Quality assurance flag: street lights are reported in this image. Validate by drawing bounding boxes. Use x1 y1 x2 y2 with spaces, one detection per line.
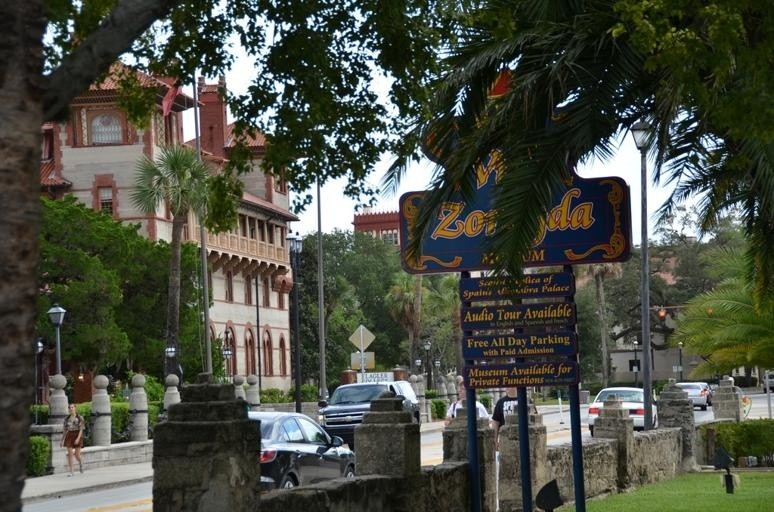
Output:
161 342 179 374
43 299 69 379
284 226 307 416
32 339 46 427
677 341 683 382
415 339 442 392
626 112 661 432
633 340 640 386
218 344 233 385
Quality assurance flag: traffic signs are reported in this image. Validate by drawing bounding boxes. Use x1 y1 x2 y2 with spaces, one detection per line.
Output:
450 276 581 390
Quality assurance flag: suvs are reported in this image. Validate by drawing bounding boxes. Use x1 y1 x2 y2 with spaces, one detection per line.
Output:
316 380 421 452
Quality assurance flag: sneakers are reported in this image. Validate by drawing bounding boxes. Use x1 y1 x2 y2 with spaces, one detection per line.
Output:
79 465 83 471
67 474 75 477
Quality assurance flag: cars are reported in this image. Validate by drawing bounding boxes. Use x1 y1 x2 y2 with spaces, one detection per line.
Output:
760 370 774 393
585 381 659 437
673 378 723 410
242 408 356 492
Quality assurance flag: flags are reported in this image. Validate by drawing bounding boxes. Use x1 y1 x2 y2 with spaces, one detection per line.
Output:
162 75 184 116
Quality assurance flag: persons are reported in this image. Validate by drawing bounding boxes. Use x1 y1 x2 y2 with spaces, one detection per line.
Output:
443 382 489 426
61 404 85 477
492 387 538 450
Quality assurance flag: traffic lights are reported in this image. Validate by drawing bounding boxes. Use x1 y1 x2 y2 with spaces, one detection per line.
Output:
658 309 668 329
